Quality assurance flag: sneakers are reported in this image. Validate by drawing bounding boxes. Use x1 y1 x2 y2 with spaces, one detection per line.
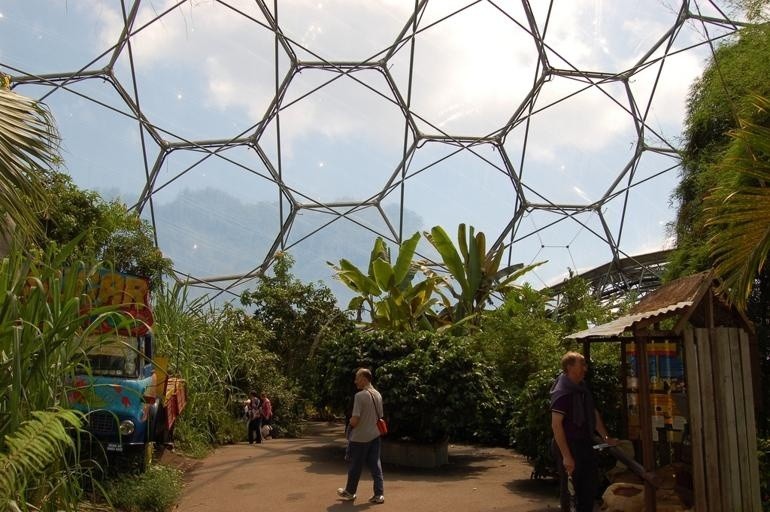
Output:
369 495 385 503
337 488 356 500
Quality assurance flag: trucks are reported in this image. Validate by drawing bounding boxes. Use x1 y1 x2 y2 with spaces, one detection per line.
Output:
16 265 189 477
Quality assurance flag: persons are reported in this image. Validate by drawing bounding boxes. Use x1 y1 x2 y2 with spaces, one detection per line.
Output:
337 367 389 503
243 391 262 445
259 391 274 440
547 352 623 511
223 384 231 409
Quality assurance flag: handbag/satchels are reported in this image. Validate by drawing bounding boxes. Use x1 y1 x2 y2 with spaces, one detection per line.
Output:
376 419 388 434
261 425 270 438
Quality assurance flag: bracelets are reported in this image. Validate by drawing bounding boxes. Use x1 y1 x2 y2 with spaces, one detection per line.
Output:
603 432 608 440
